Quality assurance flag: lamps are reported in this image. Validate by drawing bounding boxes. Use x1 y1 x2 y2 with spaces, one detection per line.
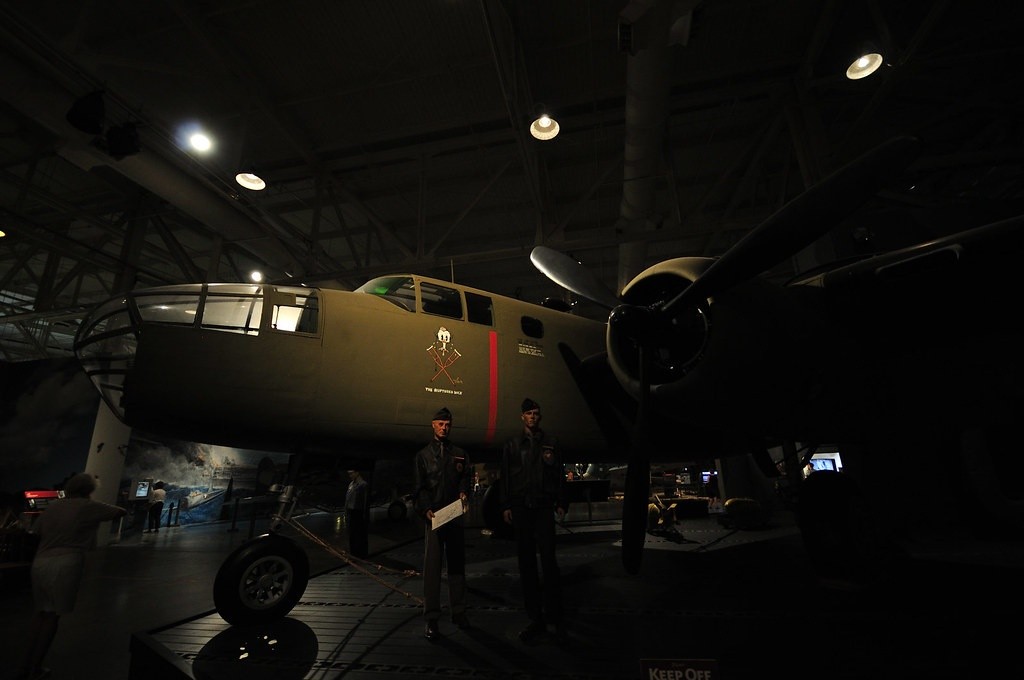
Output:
846 54 882 79
530 117 560 140
235 173 266 191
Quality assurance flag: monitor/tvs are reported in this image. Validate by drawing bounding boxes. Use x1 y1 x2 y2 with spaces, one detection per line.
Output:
810 459 836 471
702 474 709 482
136 481 150 498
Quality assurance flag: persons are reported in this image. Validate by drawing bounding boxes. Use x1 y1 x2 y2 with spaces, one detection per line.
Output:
415 406 472 639
567 463 585 481
806 463 816 477
143 481 166 533
20 472 127 680
707 468 719 509
345 465 368 562
500 397 571 645
646 497 672 529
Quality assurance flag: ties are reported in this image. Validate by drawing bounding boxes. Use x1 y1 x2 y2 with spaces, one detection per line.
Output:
440 442 445 459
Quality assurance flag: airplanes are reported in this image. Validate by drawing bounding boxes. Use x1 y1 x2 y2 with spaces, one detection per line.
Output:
0 132 1023 629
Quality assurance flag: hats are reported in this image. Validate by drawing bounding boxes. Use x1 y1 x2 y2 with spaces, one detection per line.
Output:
520 398 539 413
433 408 452 420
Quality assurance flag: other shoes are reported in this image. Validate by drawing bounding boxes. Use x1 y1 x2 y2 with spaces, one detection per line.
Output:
154 530 159 533
518 620 547 641
556 622 571 645
424 620 440 641
450 610 466 626
29 667 52 680
143 530 153 533
